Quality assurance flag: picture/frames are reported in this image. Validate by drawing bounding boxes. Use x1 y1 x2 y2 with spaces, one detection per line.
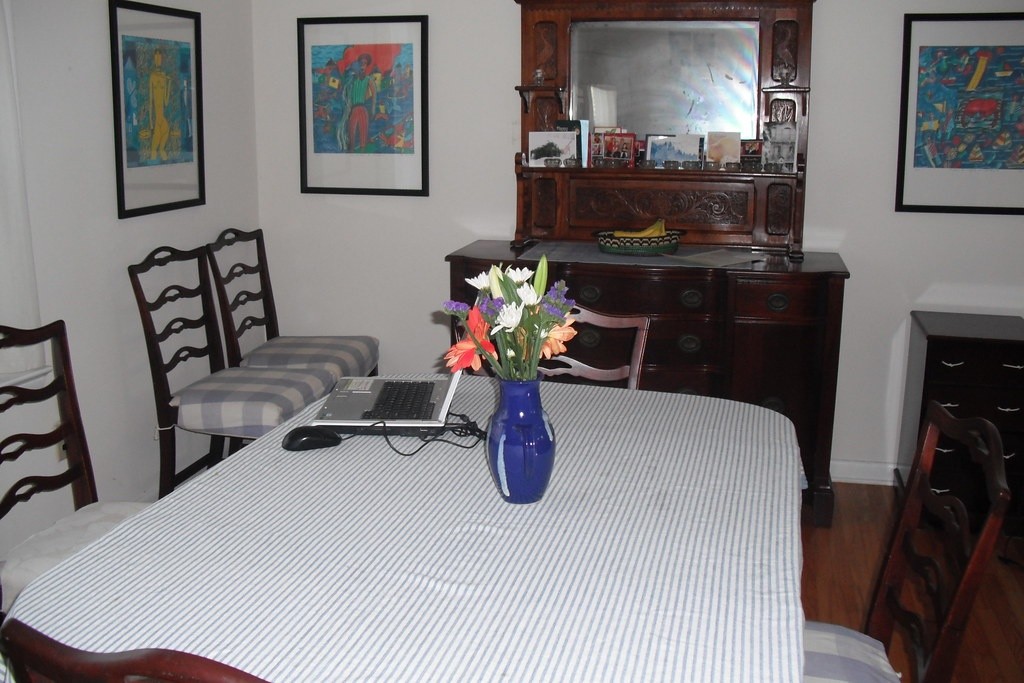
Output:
644 135 705 168
108 1 208 221
600 131 636 160
294 16 428 195
895 12 1024 219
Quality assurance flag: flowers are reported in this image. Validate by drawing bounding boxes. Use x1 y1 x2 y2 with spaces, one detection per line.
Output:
439 252 581 385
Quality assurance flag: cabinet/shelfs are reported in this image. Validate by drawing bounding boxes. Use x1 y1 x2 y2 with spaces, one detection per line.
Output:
889 310 1023 561
446 240 850 528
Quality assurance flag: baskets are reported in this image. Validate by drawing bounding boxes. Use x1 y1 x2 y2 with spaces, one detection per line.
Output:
591 227 688 257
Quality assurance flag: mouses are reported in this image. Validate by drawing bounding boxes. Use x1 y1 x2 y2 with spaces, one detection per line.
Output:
282 425 341 451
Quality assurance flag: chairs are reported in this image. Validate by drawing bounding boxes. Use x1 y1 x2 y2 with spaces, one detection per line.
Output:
538 301 649 394
803 401 1009 683
129 225 385 500
0 319 155 622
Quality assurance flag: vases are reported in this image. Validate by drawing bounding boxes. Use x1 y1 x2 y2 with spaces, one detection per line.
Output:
484 371 556 503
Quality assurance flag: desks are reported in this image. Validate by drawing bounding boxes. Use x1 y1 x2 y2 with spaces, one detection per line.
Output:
0 374 809 682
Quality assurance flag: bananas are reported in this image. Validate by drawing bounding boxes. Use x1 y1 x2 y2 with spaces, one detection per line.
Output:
612 218 667 236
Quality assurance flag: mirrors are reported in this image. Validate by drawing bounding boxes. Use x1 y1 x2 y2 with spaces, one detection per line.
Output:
571 20 760 146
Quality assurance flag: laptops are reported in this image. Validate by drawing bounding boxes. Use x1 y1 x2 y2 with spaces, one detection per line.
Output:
312 290 491 436
